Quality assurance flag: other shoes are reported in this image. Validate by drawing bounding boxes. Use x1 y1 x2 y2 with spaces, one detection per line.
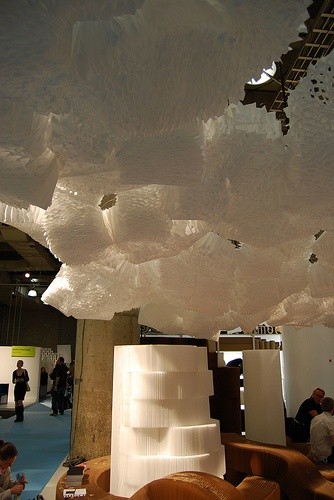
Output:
50 412 64 416
14 418 24 422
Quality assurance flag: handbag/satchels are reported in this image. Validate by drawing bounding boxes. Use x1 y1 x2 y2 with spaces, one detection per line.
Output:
26 382 30 391
63 396 72 411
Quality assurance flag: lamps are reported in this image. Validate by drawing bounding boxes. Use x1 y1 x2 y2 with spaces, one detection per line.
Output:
27 286 37 297
246 59 277 86
25 264 31 278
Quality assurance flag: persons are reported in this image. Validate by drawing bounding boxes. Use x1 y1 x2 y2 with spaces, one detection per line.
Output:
12 360 30 422
309 396 334 464
39 367 48 398
50 356 68 416
289 387 326 443
0 439 25 500
49 361 62 410
